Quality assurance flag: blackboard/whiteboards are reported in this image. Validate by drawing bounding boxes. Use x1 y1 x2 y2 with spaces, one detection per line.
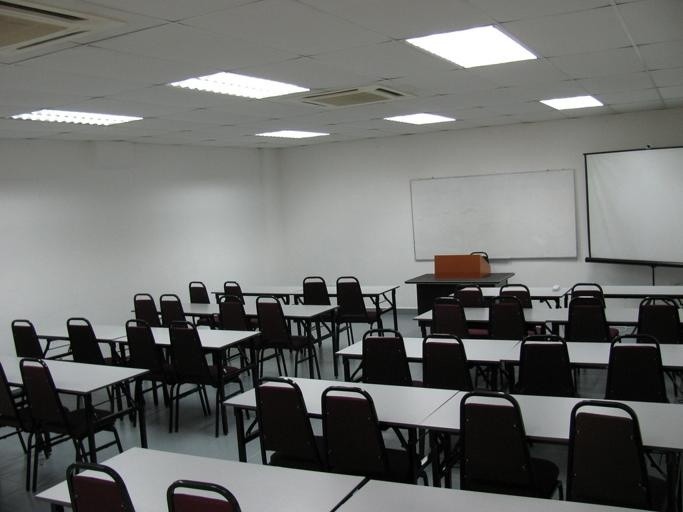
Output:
410 168 579 261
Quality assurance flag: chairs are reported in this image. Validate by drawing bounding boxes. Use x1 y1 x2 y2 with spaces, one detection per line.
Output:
4 275 680 509
